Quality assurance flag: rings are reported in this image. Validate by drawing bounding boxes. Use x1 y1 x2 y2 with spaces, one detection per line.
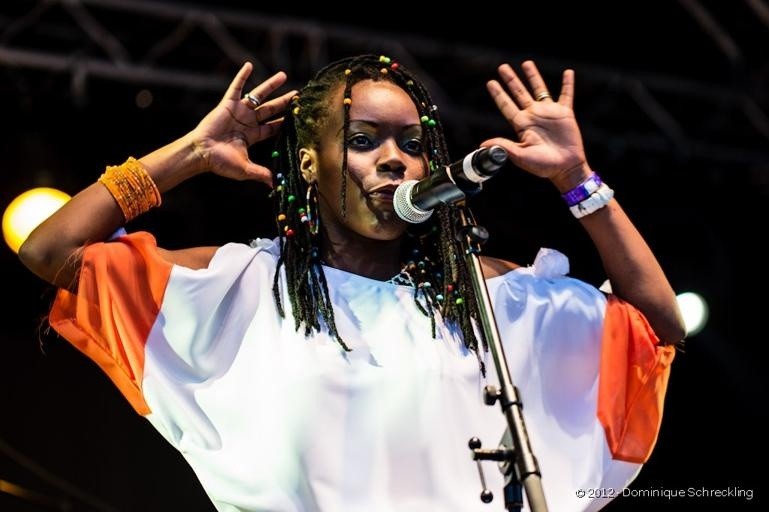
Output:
243 91 261 107
535 91 552 101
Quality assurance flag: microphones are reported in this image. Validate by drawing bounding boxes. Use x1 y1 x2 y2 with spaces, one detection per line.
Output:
392 145 508 224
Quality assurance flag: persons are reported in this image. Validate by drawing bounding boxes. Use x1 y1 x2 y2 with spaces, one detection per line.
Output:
16 55 689 512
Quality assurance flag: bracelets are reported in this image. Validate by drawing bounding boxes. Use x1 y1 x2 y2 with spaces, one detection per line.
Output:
559 169 616 220
96 151 166 223
